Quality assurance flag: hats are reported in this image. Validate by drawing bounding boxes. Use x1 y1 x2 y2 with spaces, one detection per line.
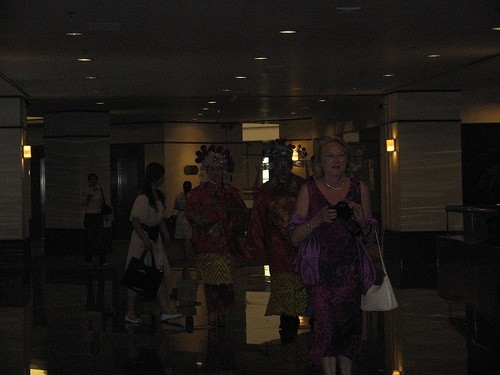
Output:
195 145 233 187
255 138 310 179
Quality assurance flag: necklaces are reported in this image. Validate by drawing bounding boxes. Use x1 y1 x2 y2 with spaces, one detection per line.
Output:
320 175 346 190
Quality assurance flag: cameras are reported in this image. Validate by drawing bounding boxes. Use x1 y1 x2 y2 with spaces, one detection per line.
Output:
329 201 353 221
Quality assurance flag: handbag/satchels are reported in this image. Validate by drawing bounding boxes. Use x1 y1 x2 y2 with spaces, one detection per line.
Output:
358 274 399 311
126 247 164 297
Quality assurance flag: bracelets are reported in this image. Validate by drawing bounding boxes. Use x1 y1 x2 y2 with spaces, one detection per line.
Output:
305 223 315 231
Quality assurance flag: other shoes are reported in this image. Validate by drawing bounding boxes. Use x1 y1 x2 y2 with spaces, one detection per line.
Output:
161 312 184 321
123 313 141 324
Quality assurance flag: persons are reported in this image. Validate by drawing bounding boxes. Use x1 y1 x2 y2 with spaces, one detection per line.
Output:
287 136 376 375
123 164 183 324
245 140 323 345
173 181 192 253
81 173 111 267
184 144 250 327
348 144 378 192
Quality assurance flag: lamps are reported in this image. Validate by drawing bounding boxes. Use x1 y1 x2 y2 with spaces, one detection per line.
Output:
23 145 32 159
386 139 395 152
